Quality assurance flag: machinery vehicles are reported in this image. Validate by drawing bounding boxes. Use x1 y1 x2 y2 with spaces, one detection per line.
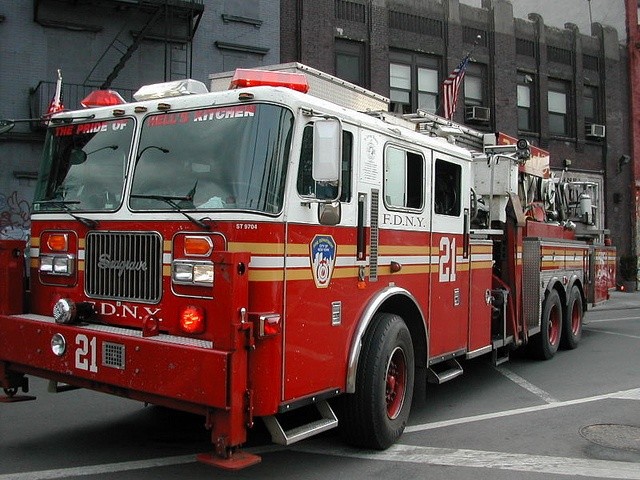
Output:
0 35 624 472
